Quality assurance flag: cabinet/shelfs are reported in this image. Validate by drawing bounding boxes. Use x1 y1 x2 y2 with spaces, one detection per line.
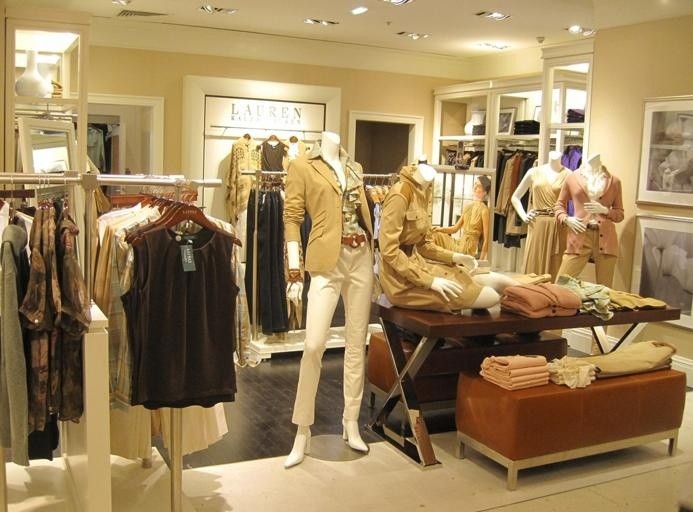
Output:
3 5 87 173
431 69 589 273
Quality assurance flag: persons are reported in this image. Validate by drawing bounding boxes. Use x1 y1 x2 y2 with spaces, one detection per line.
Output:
378 163 522 316
554 153 624 356
282 128 375 468
510 151 573 281
429 175 490 261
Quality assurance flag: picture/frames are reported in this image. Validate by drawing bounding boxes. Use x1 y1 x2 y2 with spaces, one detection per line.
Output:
628 95 693 330
497 107 517 135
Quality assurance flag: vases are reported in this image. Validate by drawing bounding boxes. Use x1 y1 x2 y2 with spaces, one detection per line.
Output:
15 50 50 98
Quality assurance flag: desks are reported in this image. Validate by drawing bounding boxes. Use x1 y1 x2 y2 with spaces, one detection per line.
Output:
363 292 681 472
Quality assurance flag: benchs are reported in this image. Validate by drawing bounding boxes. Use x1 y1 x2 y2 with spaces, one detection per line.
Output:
365 332 569 431
456 351 685 487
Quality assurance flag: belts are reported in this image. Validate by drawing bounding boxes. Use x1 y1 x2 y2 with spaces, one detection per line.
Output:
587 223 600 231
342 234 369 250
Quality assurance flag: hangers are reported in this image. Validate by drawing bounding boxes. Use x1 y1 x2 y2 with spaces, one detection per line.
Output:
123 182 242 250
6 174 71 233
242 129 297 144
